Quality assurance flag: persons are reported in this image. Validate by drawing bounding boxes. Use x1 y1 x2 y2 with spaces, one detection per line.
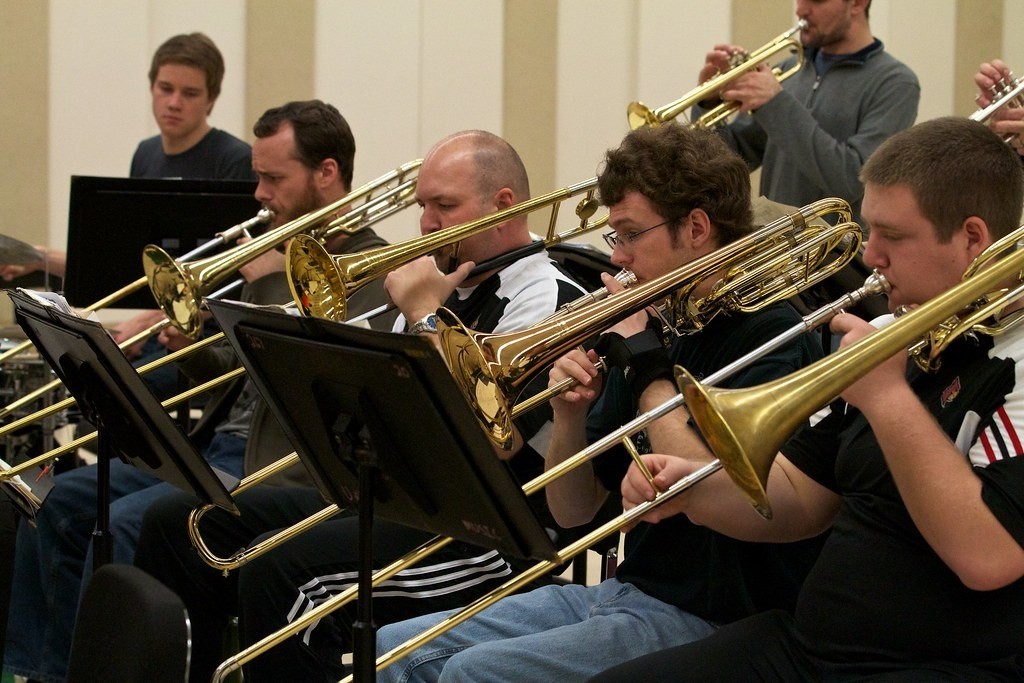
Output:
1 99 409 683
589 116 1024 683
377 119 828 681
228 127 613 682
691 0 923 322
2 31 260 288
973 56 1024 161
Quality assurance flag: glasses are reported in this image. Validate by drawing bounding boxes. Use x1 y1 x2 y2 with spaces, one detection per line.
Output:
603 214 688 250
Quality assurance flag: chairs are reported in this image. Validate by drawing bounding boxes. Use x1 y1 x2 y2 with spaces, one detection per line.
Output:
64 562 192 683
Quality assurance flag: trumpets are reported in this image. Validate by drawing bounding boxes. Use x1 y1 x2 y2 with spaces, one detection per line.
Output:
967 69 1024 145
626 20 809 132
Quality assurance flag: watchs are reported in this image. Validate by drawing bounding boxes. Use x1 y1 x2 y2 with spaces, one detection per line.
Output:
407 314 437 335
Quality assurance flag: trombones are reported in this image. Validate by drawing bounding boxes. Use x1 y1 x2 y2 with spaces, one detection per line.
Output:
0 175 611 480
0 155 424 420
210 219 1023 683
187 196 864 578
